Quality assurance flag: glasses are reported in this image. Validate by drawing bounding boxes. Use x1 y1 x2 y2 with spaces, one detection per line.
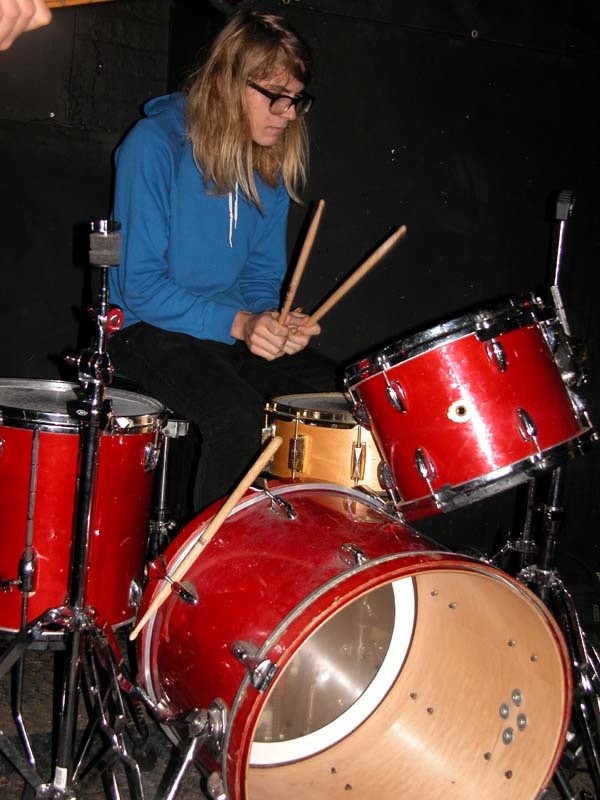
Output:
246 79 313 116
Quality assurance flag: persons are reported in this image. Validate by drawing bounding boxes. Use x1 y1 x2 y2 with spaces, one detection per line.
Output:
106 9 333 515
0 0 175 375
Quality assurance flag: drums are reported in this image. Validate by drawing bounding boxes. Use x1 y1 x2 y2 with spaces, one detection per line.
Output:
130 478 574 800
343 291 600 525
260 393 397 507
0 376 171 638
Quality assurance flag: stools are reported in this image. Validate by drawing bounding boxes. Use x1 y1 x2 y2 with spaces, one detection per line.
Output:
106 372 191 561
